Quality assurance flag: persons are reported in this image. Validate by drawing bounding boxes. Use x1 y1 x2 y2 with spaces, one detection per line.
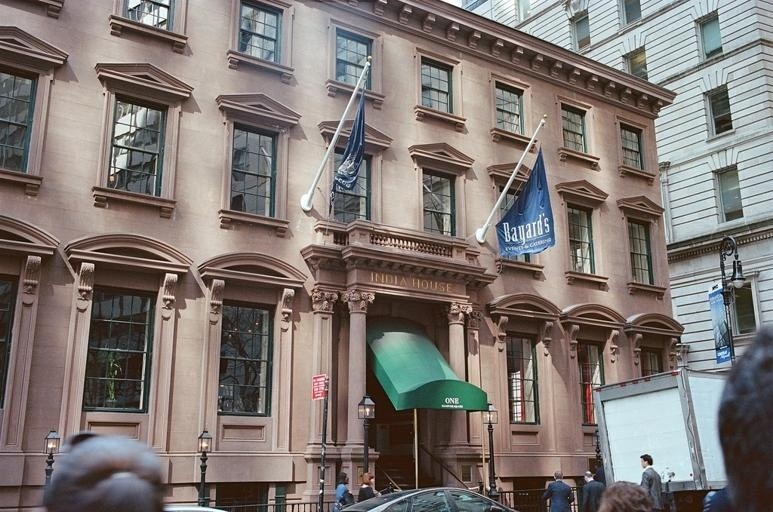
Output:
701 484 732 512
540 470 574 512
638 454 665 512
580 471 605 511
39 433 168 512
333 472 351 512
714 324 772 512
598 480 651 512
356 471 378 504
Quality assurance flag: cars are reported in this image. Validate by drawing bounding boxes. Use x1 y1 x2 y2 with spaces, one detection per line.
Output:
335 484 520 512
162 503 228 511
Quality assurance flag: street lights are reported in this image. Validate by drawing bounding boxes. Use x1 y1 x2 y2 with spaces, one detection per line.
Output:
479 401 499 506
41 426 61 508
717 233 745 368
355 393 377 500
195 430 213 507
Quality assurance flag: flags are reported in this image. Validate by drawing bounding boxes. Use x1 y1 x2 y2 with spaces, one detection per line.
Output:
493 144 555 259
328 81 367 218
